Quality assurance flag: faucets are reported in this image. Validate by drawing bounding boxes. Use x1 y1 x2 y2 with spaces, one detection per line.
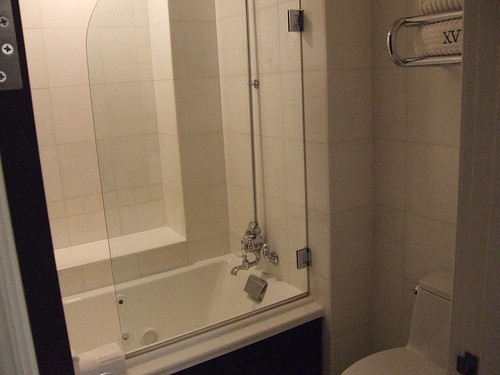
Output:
231 254 261 275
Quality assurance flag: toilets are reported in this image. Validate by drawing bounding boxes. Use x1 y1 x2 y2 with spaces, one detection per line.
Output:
340 262 453 375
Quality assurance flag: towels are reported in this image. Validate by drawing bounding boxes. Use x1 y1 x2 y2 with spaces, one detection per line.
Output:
70 342 127 375
410 0 464 57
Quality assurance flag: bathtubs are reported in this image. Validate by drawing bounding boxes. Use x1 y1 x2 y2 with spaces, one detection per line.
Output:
60 253 307 363
